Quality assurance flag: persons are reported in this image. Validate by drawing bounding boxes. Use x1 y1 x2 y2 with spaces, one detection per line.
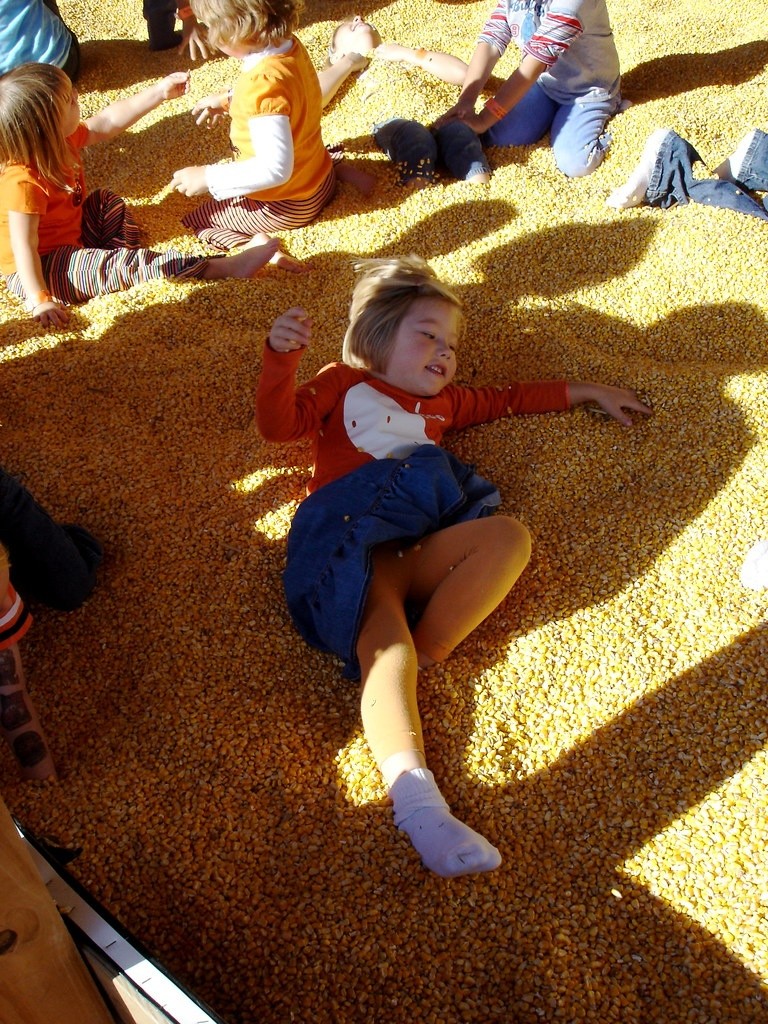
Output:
0 468 100 783
254 254 654 877
0 0 768 328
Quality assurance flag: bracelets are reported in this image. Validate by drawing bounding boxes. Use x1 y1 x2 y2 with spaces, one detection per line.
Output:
484 98 507 120
